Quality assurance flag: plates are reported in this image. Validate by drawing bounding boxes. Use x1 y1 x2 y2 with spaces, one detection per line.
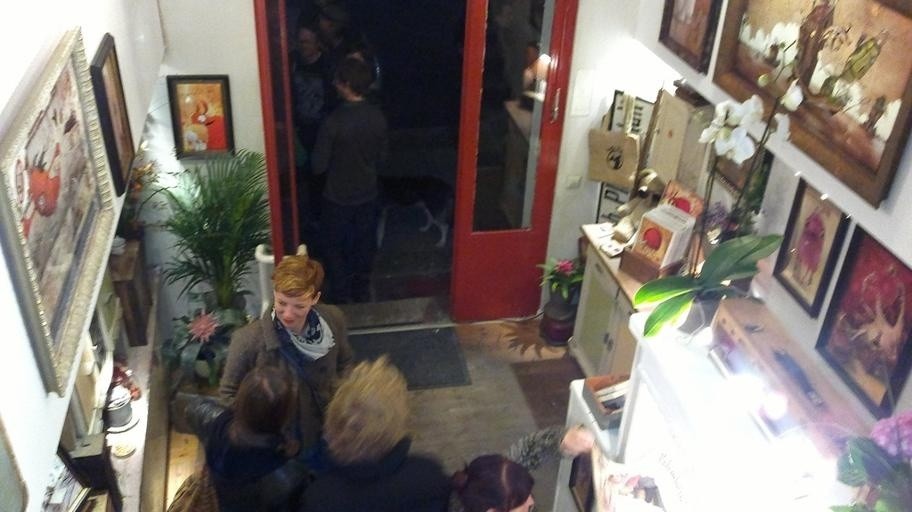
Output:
102 413 139 435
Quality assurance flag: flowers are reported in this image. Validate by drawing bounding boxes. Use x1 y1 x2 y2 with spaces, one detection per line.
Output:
127 140 161 203
160 303 248 390
828 407 912 512
535 253 587 309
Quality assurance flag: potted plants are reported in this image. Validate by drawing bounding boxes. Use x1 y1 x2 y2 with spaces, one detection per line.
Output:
147 144 277 318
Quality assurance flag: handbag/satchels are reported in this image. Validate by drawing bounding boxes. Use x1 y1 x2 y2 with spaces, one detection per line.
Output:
165 472 220 511
587 95 640 193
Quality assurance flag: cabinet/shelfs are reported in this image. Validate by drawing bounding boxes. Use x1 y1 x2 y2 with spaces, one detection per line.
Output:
109 220 155 348
565 222 648 383
499 101 534 229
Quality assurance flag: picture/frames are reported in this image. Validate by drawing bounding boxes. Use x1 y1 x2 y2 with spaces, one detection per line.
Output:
87 31 139 202
566 423 596 511
164 70 239 159
1 24 119 396
0 417 29 512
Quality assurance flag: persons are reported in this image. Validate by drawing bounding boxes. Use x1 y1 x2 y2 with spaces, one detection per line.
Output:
171 366 312 512
451 423 594 511
220 255 356 427
278 0 542 303
301 352 447 510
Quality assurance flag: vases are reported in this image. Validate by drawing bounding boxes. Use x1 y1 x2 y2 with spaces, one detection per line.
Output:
540 299 576 346
165 381 226 435
121 196 145 240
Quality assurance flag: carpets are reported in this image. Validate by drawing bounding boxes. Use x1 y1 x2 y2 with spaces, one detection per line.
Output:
343 322 474 392
510 353 588 430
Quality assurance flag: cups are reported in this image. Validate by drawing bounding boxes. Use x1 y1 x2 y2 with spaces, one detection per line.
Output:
109 235 127 255
104 388 133 427
